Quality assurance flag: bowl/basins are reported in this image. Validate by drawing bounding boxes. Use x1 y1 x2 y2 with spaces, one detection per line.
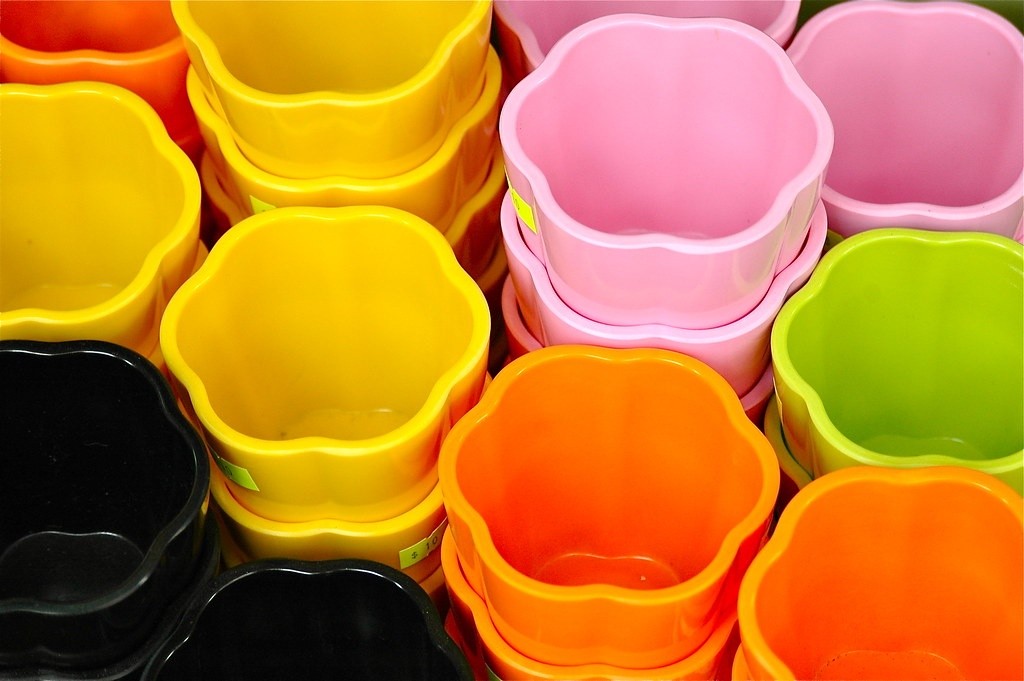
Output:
479 234 506 294
733 644 757 681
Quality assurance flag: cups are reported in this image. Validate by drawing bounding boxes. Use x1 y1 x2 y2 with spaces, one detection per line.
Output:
439 344 780 669
772 228 1024 494
0 512 222 681
202 149 502 248
171 372 491 582
170 0 490 178
496 189 827 397
440 524 740 681
0 0 197 163
160 208 491 521
139 559 474 681
0 341 209 653
0 81 206 356
500 14 834 328
502 274 774 422
764 397 812 490
494 0 799 67
737 467 1024 681
214 514 446 602
785 0 1024 244
187 46 501 207
